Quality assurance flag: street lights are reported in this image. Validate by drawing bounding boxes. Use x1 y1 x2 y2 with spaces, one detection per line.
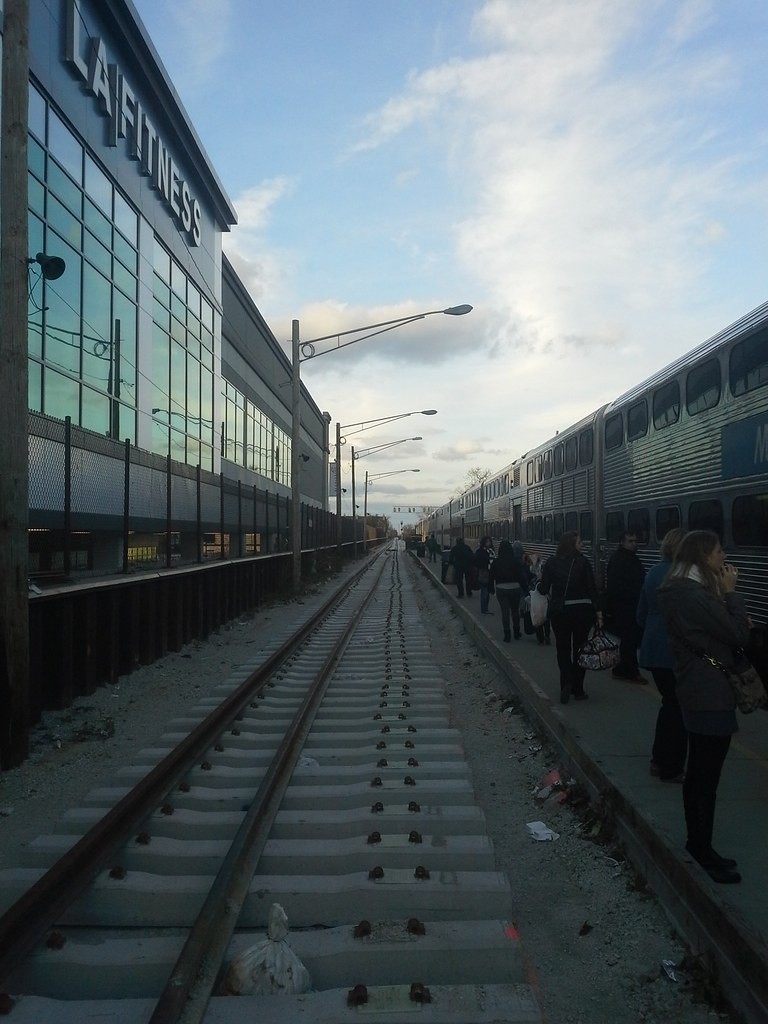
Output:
292 306 474 600
351 437 423 561
363 469 420 555
335 408 438 571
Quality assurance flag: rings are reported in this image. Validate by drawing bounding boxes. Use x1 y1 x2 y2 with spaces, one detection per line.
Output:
732 572 737 575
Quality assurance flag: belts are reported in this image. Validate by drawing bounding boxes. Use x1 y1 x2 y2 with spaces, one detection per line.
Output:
565 599 592 605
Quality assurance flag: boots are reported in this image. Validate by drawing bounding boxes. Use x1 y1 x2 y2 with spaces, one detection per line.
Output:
682 783 740 884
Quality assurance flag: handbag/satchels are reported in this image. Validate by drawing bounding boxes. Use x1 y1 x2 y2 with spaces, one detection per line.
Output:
578 626 621 671
529 583 548 627
524 602 535 634
727 663 767 715
446 563 455 583
546 591 565 616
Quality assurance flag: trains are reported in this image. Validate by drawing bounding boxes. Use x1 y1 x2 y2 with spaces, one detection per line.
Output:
415 302 768 651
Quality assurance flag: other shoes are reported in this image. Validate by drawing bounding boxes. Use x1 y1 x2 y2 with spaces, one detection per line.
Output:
515 633 522 640
574 692 590 700
482 611 494 616
504 637 510 643
611 668 649 685
561 695 569 703
651 763 686 783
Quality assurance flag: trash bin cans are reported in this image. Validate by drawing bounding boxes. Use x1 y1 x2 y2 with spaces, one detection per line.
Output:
417 541 425 558
440 549 456 586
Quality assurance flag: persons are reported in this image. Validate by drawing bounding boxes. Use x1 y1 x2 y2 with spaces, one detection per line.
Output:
449 532 648 706
637 528 749 885
425 534 437 563
